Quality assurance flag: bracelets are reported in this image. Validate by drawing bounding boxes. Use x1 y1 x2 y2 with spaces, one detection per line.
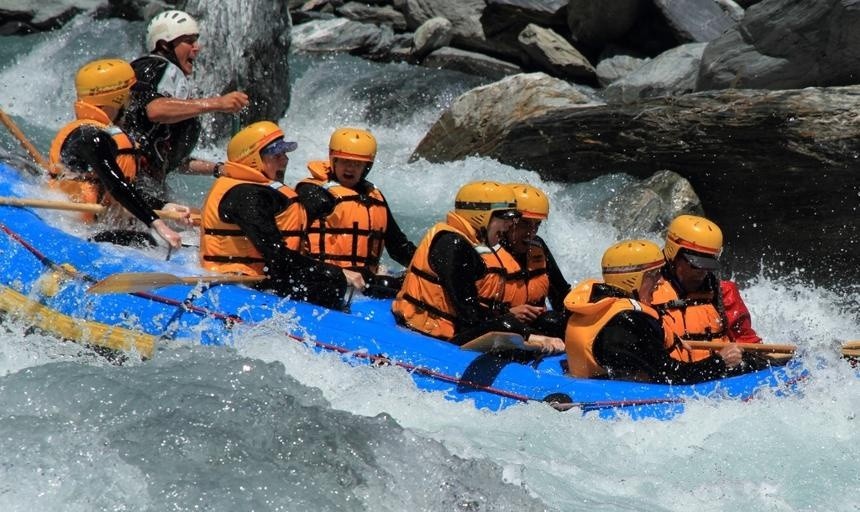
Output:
212 162 225 177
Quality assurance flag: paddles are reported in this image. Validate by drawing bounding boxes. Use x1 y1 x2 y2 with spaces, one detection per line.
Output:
85 273 266 293
460 331 543 351
684 340 860 356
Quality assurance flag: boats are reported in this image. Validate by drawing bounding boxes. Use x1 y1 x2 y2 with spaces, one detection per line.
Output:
0 154 815 423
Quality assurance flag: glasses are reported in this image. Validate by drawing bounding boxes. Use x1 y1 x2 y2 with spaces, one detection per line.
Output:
647 269 661 281
178 36 197 44
516 219 541 231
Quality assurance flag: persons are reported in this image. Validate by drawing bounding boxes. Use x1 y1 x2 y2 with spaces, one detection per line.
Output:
117 9 249 231
293 125 417 297
201 121 347 308
648 213 786 378
491 182 572 341
47 58 195 266
391 181 564 365
561 240 742 388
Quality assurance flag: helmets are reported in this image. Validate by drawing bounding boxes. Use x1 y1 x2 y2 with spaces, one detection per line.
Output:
146 10 199 52
75 59 137 109
601 215 723 293
455 182 549 231
227 120 297 172
329 128 376 178
